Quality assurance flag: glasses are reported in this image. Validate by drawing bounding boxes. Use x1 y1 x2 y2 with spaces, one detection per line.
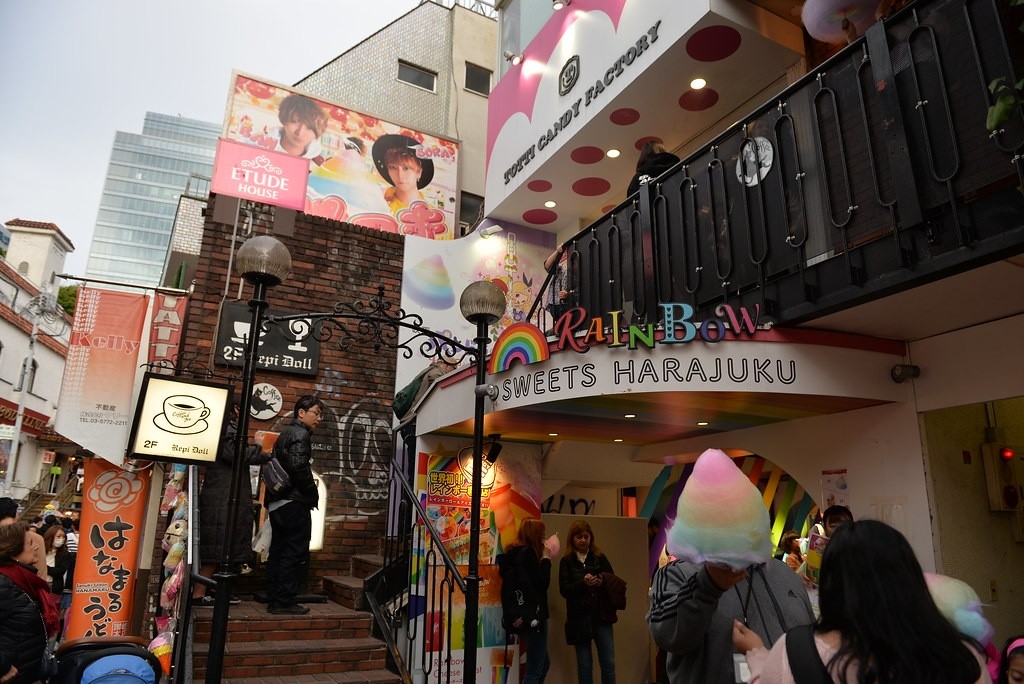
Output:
304 408 323 420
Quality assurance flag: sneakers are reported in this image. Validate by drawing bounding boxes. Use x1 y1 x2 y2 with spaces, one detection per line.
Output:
192 596 215 609
205 588 241 604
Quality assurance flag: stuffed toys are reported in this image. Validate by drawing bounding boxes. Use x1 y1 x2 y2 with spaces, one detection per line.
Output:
148 462 188 676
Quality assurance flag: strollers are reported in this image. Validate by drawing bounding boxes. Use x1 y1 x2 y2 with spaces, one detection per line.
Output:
51 635 162 684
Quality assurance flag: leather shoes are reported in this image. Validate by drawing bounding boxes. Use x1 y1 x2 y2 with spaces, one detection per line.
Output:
267 600 311 614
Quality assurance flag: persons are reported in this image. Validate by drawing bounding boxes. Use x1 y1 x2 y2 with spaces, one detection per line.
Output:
841 0 914 45
196 377 275 607
262 394 325 616
744 119 772 143
558 519 628 684
995 634 1024 684
498 516 552 684
542 242 583 325
780 530 807 573
626 141 681 223
645 551 819 684
797 504 856 622
0 497 80 684
236 93 446 236
647 514 662 551
731 519 992 684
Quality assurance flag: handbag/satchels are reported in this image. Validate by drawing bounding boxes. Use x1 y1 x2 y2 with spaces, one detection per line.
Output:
564 614 593 645
260 428 293 494
501 547 549 633
42 652 59 675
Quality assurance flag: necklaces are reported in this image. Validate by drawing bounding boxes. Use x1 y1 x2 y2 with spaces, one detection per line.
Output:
734 564 754 629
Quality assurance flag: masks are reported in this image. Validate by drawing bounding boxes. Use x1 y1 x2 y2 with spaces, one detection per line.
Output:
52 537 65 548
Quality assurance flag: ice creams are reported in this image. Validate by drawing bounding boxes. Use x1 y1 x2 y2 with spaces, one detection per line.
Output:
149 637 172 675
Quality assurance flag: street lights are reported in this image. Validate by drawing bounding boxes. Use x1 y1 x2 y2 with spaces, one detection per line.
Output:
460 280 507 684
203 234 293 684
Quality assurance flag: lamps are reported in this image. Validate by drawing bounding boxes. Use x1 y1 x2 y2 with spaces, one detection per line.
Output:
552 0 571 10
487 433 503 462
504 50 524 66
475 383 498 400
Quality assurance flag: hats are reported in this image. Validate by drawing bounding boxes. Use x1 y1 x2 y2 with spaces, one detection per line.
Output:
371 134 434 191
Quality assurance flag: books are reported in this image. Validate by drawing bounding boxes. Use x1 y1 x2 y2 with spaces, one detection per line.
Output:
805 532 832 591
